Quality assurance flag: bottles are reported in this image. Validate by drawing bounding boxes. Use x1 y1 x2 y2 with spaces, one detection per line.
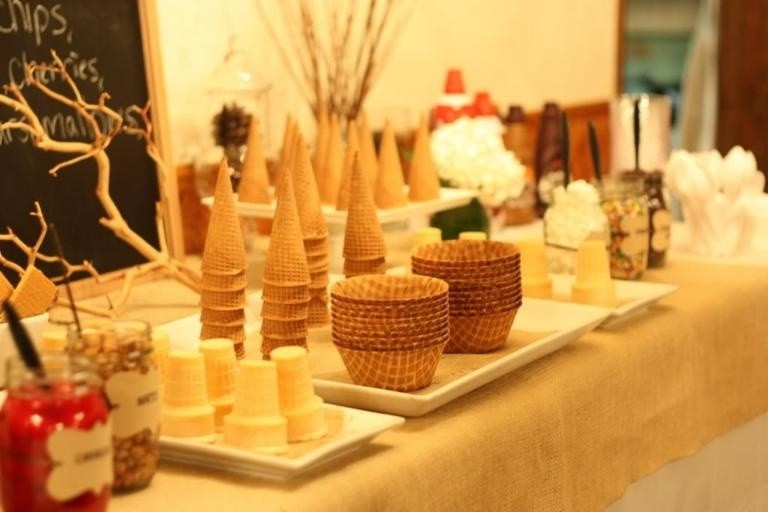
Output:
536 103 566 218
1 351 113 510
504 106 536 226
64 317 162 494
544 173 672 280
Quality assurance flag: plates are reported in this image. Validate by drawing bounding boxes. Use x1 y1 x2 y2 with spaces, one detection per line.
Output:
158 399 406 480
522 274 678 328
201 187 475 223
153 272 609 418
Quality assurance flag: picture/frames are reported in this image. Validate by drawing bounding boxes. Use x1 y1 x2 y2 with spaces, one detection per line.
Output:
1 2 187 318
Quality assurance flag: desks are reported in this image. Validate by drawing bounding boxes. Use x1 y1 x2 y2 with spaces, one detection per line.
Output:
203 186 476 247
